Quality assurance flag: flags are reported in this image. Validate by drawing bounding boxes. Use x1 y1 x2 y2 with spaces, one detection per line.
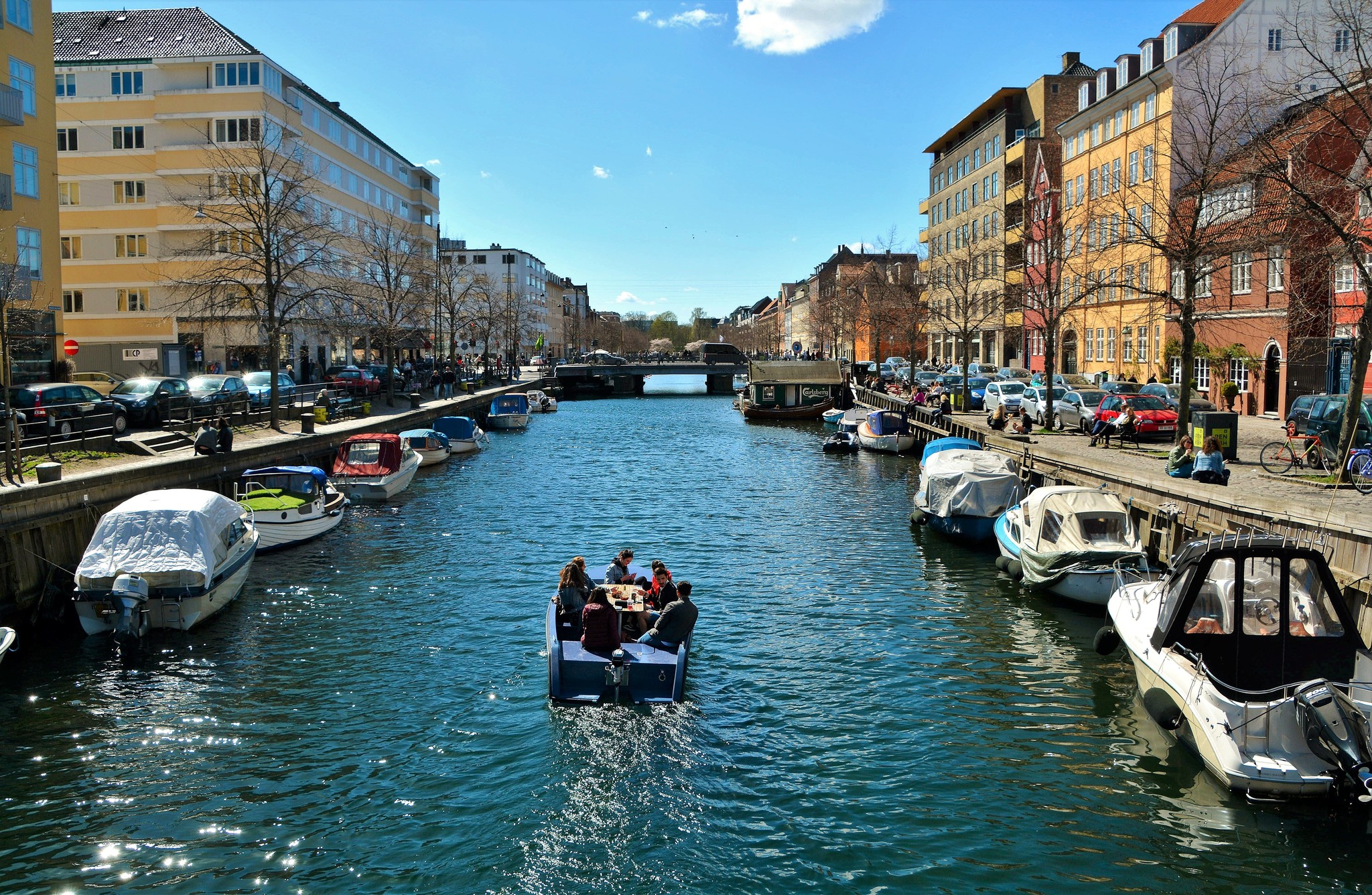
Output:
535 334 542 350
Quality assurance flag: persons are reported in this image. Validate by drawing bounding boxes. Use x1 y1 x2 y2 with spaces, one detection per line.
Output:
558 549 698 652
638 348 692 365
1117 373 1126 381
931 354 937 366
402 352 526 399
1146 372 1157 384
540 350 598 366
1165 435 1194 478
904 380 952 428
1013 406 1032 435
936 358 963 374
1085 404 1138 449
988 403 1012 432
194 416 233 456
1202 392 1208 401
1192 435 1231 486
318 389 336 420
286 359 324 384
1042 376 1046 385
1128 373 1137 383
755 348 827 361
862 375 889 399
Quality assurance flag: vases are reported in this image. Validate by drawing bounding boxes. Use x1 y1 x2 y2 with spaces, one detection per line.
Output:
77 404 96 412
49 417 55 426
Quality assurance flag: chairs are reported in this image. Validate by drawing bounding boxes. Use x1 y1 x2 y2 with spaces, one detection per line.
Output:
1329 409 1340 421
1077 394 1090 405
1289 620 1311 636
1186 618 1226 635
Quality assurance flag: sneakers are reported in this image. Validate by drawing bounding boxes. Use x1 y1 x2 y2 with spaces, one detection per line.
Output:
623 622 637 631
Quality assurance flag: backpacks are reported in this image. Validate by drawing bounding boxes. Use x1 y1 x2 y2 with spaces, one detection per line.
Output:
987 411 994 425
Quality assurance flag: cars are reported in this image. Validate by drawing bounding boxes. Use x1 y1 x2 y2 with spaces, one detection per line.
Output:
833 356 1217 443
187 364 252 419
333 369 381 397
556 359 567 365
241 371 296 411
530 356 543 366
583 353 626 366
106 376 195 428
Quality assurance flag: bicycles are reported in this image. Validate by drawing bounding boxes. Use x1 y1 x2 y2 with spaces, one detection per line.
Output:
501 363 508 372
473 362 485 371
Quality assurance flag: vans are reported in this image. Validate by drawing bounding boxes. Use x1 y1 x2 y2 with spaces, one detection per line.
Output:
700 342 748 365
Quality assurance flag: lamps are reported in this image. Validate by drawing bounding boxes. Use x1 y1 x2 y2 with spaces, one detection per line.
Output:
801 285 805 292
557 302 561 307
785 298 789 302
1122 324 1132 334
453 269 458 276
193 188 207 219
540 291 545 297
372 242 379 256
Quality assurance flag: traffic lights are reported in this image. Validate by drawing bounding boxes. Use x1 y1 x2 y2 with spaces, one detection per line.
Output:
454 340 459 348
591 338 599 346
495 341 499 348
468 339 476 347
516 340 519 349
471 322 474 328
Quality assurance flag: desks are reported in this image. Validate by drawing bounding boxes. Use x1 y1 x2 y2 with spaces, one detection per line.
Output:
600 583 643 638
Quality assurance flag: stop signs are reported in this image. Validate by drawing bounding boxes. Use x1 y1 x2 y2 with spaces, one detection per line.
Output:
63 340 79 356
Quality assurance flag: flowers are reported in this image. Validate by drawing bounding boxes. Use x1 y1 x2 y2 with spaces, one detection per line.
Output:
49 413 56 417
82 403 92 406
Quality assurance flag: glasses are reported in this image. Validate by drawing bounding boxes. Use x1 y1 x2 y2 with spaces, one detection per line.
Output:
627 551 634 555
1185 441 1192 444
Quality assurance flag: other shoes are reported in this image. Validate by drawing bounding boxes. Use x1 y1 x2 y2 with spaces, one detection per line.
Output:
1085 431 1093 438
1087 443 1096 447
1102 445 1109 449
1090 435 1099 440
932 421 937 425
938 425 942 429
908 414 912 418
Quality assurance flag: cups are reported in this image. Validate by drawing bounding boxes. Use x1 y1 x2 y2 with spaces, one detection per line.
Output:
1189 452 1195 462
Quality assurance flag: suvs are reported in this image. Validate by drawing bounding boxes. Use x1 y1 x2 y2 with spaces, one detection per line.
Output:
0 383 127 448
323 364 360 387
67 371 127 397
356 364 406 393
1260 393 1372 494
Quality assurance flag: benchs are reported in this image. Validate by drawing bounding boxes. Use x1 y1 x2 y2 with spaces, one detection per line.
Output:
314 396 363 421
1096 415 1143 448
461 377 481 390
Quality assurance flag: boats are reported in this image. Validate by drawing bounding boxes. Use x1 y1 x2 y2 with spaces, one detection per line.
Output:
330 416 481 499
822 405 915 454
486 390 557 429
71 487 261 637
227 466 351 550
912 437 1372 805
547 578 693 703
0 627 16 665
732 358 843 418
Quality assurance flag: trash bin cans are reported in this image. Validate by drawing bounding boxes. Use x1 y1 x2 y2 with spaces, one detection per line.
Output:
1191 412 1239 460
950 383 972 410
